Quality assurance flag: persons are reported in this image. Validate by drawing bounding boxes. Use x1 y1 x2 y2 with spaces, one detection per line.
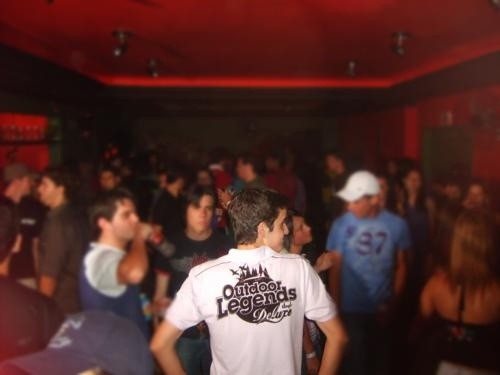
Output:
325 170 412 374
284 207 338 375
0 195 62 375
78 187 162 340
1 142 499 283
0 308 157 375
156 184 234 375
409 209 500 373
149 187 350 375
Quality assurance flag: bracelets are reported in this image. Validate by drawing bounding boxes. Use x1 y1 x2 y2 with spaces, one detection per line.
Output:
305 351 317 359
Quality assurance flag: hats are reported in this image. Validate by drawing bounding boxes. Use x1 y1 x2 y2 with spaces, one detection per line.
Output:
6 310 153 375
336 171 379 203
5 161 40 182
